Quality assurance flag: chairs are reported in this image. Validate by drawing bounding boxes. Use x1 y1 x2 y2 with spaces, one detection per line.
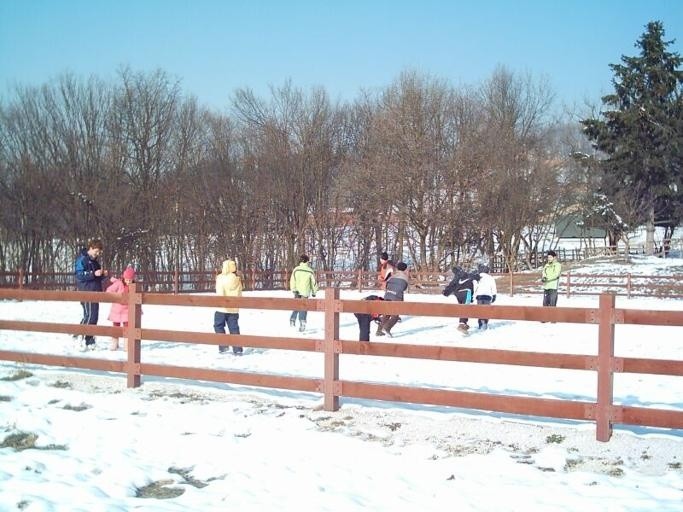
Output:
375 331 385 336
383 327 393 337
88 343 96 350
219 347 229 353
457 324 469 336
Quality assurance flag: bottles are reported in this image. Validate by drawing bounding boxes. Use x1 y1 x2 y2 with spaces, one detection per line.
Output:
124 268 135 281
222 260 237 274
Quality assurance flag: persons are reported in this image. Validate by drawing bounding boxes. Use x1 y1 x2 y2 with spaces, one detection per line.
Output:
472 265 497 329
377 252 402 322
539 251 562 324
376 262 409 337
442 266 482 337
72 239 108 352
354 295 384 341
289 254 320 332
104 264 142 351
214 260 244 354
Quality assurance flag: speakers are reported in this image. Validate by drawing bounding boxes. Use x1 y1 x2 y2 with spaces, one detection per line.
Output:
290 319 295 326
299 320 307 333
123 337 129 352
109 338 119 350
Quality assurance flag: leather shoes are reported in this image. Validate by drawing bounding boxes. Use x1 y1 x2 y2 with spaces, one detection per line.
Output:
492 295 495 302
